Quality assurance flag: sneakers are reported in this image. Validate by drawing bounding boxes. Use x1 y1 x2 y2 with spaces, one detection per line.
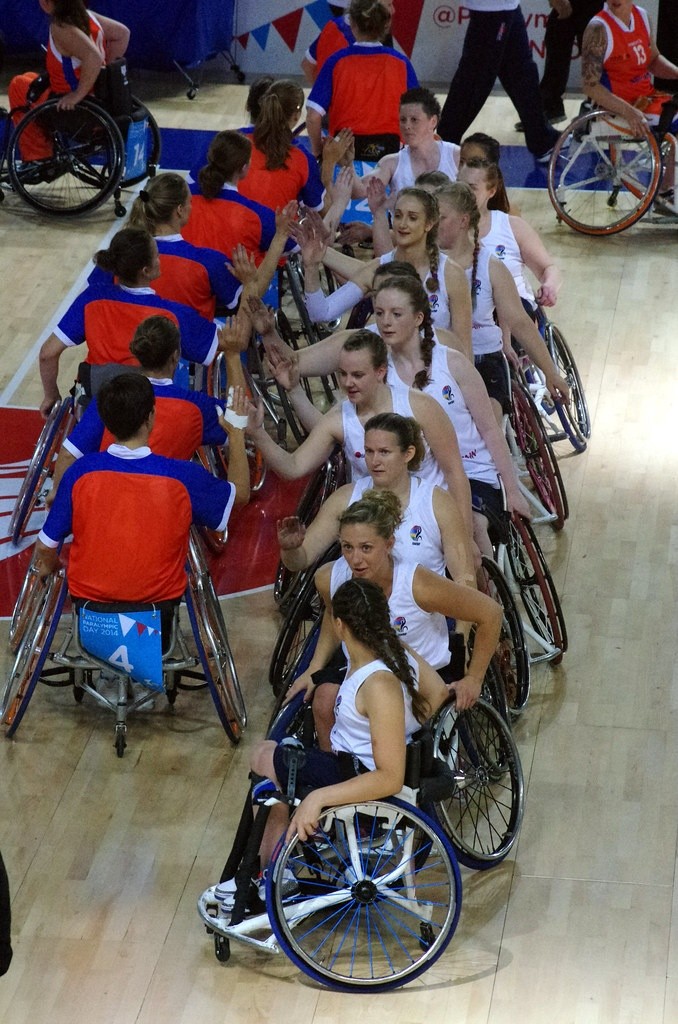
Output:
537 134 575 162
222 871 300 912
213 871 273 900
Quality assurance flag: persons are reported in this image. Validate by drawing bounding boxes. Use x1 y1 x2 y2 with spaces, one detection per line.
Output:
513 0 608 131
437 0 564 167
9 0 130 170
36 71 354 656
213 89 576 919
579 0 678 204
298 1 424 160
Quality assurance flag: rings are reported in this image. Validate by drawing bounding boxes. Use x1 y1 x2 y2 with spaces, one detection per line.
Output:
334 136 340 142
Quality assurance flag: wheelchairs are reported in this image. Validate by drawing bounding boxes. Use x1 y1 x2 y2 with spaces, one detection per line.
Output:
547 96 677 238
202 285 593 994
0 59 162 216
0 189 343 745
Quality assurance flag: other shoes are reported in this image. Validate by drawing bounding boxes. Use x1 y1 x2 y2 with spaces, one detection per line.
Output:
514 104 567 131
